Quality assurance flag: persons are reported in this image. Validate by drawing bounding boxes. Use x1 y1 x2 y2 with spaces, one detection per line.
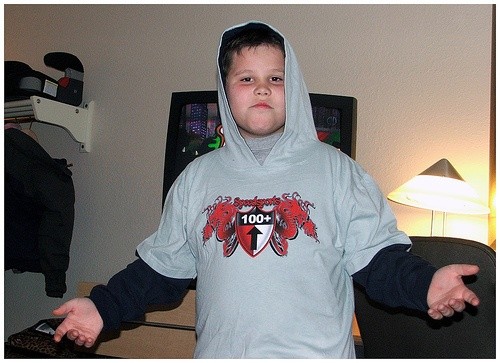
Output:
53 20 479 359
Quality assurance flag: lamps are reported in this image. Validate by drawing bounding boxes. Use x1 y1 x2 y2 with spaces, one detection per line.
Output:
388 158 491 235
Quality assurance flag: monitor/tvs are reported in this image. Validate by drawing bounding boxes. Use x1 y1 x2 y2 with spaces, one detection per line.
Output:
162 90 358 213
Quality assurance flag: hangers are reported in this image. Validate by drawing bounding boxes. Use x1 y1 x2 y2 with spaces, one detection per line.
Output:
4 114 38 144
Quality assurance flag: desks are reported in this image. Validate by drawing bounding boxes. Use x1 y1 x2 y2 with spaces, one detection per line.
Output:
80 281 363 359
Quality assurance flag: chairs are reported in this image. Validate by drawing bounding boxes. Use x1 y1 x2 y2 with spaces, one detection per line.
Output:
353 236 496 357
